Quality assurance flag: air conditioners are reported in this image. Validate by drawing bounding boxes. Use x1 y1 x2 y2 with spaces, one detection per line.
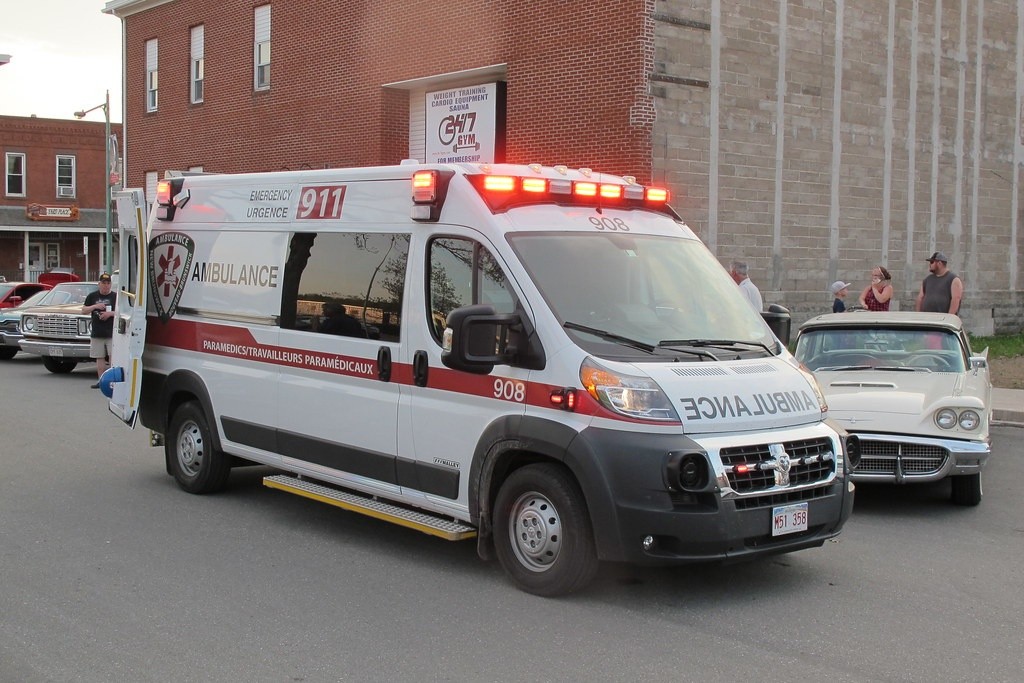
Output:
58 186 75 196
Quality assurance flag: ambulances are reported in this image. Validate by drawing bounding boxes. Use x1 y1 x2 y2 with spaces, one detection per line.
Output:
109 150 863 599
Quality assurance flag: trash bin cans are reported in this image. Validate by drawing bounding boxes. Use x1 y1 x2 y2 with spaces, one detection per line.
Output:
759 303 792 351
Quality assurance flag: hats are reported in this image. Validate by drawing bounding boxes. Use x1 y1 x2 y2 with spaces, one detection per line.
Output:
100 273 111 281
877 264 891 280
832 281 851 295
926 252 948 262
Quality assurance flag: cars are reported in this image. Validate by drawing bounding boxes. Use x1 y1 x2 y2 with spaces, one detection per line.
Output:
779 309 994 504
17 280 115 375
0 289 89 359
0 271 80 308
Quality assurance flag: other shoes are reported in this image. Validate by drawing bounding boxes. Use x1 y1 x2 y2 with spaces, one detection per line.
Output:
91 382 100 389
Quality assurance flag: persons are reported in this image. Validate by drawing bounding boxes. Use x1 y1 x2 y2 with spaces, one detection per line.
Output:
917 252 964 348
831 280 852 350
727 259 763 313
311 302 363 338
81 274 118 388
859 266 893 350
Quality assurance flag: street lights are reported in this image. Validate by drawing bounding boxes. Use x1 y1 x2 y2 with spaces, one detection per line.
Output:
74 88 113 282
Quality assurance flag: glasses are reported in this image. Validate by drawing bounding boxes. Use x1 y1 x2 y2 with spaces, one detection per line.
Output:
930 261 935 264
